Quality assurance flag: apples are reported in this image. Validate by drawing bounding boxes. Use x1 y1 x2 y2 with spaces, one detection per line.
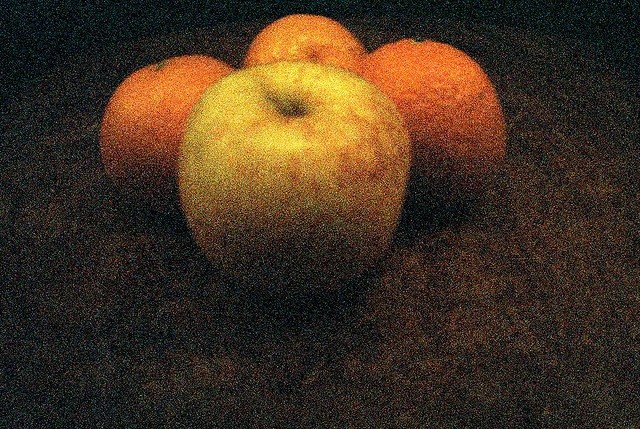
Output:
179 59 413 295
